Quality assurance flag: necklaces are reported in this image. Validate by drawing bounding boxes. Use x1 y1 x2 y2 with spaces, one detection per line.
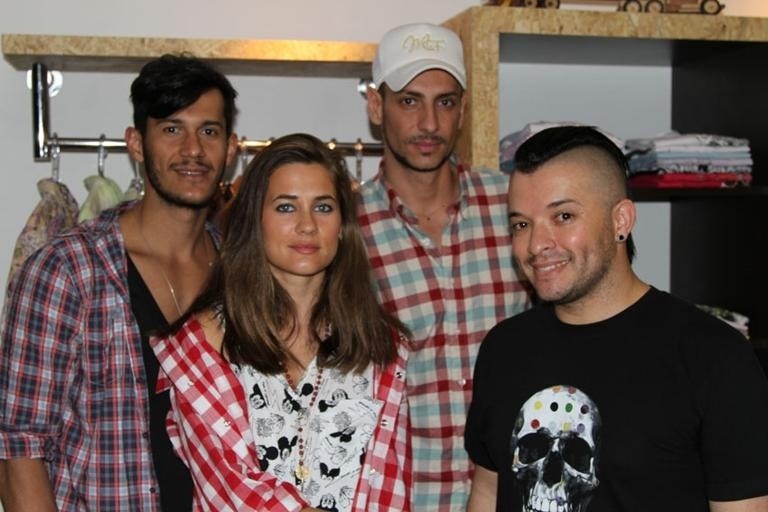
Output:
412 205 449 222
279 317 334 492
132 208 213 319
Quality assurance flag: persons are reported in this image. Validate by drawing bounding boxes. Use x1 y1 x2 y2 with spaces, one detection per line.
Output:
463 129 767 512
149 131 418 512
202 20 542 512
0 50 239 512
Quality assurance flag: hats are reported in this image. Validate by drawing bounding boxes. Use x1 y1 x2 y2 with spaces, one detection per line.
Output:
371 21 467 93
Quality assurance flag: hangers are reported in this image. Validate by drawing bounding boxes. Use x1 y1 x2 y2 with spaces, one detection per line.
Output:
83 132 123 189
48 132 63 189
232 135 252 188
350 136 365 188
129 156 146 189
